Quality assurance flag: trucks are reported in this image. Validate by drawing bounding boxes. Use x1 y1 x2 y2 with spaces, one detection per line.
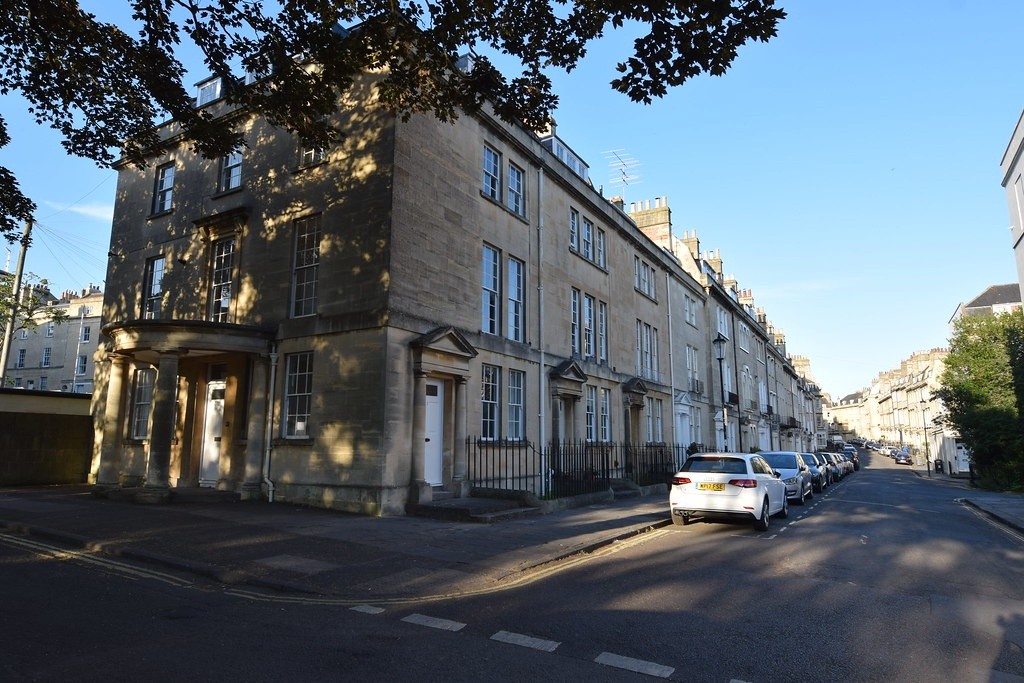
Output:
832 435 843 444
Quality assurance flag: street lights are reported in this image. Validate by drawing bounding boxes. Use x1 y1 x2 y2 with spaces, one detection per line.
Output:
712 333 729 452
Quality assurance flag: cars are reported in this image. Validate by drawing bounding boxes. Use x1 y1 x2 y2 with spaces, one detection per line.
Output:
819 452 854 483
850 438 900 458
669 450 788 532
841 443 860 471
799 453 827 493
895 452 912 465
755 452 814 505
